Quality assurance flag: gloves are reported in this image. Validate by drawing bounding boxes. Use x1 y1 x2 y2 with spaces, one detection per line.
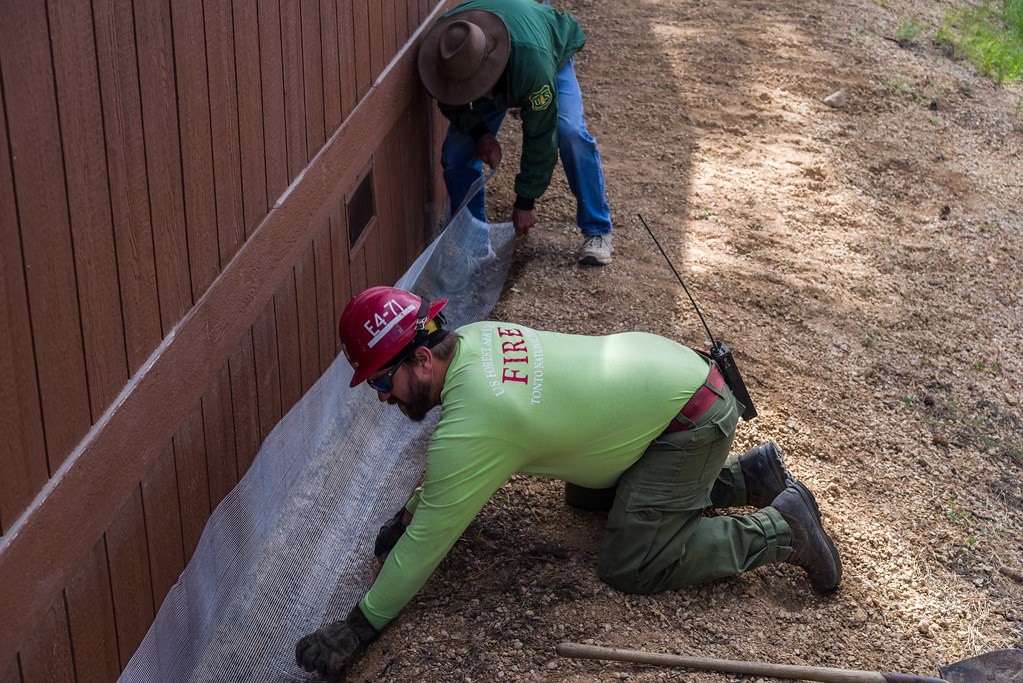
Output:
374 507 407 566
295 602 380 683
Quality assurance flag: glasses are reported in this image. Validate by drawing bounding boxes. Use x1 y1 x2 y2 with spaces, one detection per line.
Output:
366 353 412 393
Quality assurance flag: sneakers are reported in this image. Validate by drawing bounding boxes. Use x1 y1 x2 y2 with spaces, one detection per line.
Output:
738 440 796 509
436 241 495 293
770 478 843 598
577 233 613 265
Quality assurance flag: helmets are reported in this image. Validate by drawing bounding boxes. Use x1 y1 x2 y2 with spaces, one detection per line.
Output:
338 286 449 387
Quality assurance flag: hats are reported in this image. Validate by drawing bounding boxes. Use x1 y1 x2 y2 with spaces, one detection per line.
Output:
416 9 511 105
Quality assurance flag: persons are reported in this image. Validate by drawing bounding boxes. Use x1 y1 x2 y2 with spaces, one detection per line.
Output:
418 0 612 292
296 287 842 683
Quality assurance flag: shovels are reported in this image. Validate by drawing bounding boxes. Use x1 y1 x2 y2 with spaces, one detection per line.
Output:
555 641 1023 683
